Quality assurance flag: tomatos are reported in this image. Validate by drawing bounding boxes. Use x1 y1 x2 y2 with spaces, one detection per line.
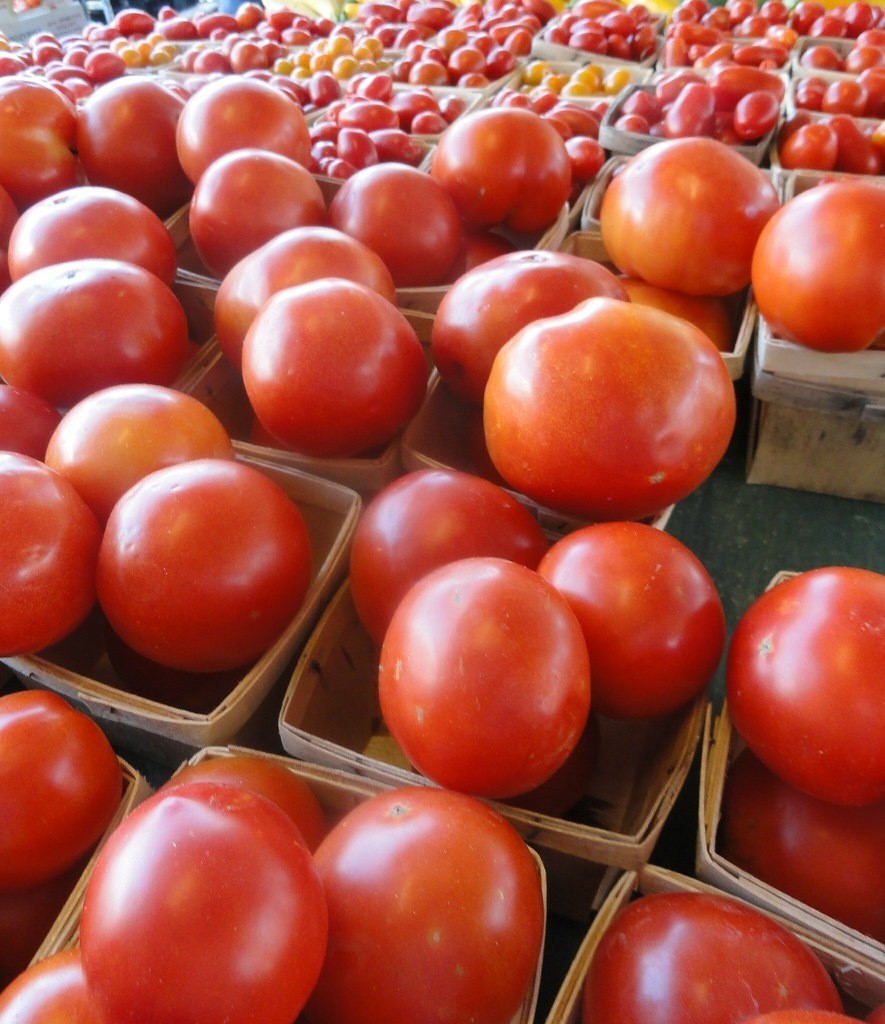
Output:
0 0 885 1024
